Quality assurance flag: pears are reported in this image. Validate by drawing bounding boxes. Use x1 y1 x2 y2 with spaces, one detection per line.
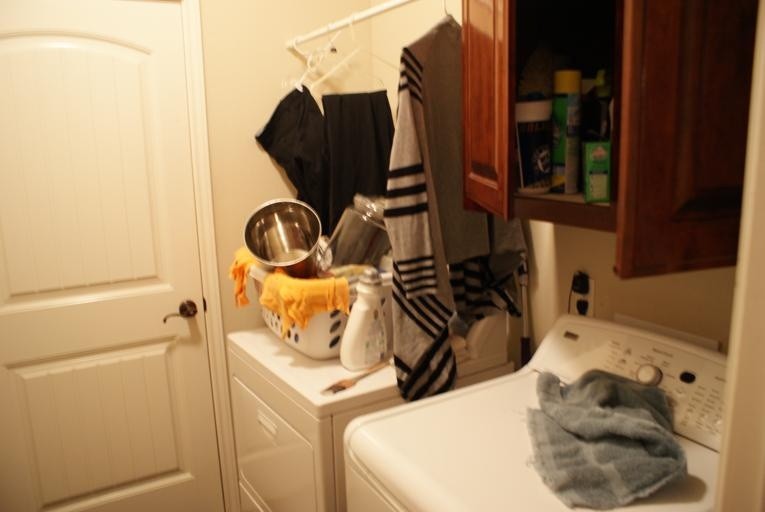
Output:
338 268 391 371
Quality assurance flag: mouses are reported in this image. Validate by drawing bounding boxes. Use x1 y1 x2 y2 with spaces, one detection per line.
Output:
434 0 450 22
289 23 402 102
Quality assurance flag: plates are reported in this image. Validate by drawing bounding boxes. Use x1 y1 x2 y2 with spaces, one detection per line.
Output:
463 0 757 280
224 326 515 511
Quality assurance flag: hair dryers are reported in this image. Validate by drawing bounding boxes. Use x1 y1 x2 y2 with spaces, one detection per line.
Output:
339 309 731 512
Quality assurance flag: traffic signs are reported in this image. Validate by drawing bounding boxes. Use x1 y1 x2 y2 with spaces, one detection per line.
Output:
571 273 596 315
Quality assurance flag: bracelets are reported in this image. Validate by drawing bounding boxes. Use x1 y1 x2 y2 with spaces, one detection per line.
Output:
245 200 325 276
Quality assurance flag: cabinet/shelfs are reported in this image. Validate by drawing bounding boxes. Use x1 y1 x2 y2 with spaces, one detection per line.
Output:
586 70 610 140
511 98 552 194
317 193 395 280
